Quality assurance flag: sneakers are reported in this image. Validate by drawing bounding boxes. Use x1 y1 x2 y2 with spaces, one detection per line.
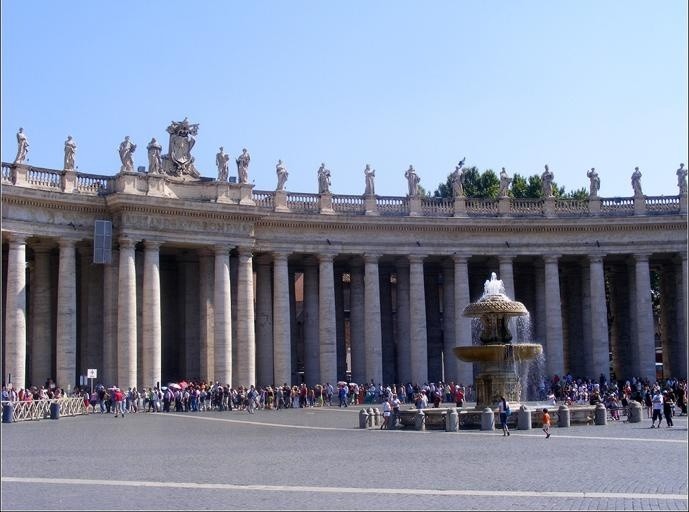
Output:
546 433 551 439
504 431 510 436
651 424 676 428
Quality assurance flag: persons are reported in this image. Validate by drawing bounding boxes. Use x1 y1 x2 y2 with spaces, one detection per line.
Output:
542 407 551 438
498 396 510 436
650 389 663 427
542 164 553 197
16 128 29 162
337 379 464 409
379 397 391 430
317 164 331 194
587 168 600 195
537 375 687 416
216 146 250 182
500 167 510 196
64 135 76 168
364 164 375 195
405 165 420 197
450 166 463 195
631 167 643 197
119 136 162 173
276 160 288 190
2 377 335 417
676 163 687 193
661 391 674 428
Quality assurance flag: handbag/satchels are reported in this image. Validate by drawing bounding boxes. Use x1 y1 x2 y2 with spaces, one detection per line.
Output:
506 408 511 417
